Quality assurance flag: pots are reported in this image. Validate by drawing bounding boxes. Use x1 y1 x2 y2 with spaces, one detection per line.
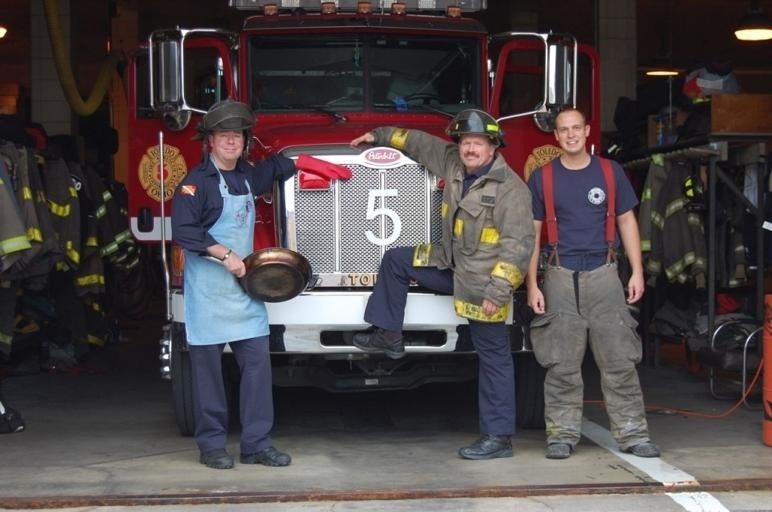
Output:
199 248 313 301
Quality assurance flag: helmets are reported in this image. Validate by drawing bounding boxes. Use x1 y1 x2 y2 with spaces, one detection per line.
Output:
447 110 505 150
197 98 253 156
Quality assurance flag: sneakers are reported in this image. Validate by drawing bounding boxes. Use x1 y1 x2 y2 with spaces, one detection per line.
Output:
630 442 662 457
459 435 514 458
199 448 235 468
547 443 573 457
241 447 292 467
354 331 407 360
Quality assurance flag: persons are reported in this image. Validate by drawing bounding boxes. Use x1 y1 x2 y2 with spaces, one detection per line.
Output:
525 107 659 459
171 101 352 470
350 109 536 460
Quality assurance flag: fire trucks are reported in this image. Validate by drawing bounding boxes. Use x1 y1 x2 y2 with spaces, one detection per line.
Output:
128 0 605 439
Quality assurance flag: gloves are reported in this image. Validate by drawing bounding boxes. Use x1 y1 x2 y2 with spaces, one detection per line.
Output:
295 153 352 180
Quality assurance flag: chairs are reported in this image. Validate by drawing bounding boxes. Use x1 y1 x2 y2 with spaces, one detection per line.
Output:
386 69 439 105
698 318 763 412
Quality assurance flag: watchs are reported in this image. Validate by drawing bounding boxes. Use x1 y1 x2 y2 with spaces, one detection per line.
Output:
221 249 232 262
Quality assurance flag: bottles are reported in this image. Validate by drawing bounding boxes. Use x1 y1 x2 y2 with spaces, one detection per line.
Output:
655 117 664 147
